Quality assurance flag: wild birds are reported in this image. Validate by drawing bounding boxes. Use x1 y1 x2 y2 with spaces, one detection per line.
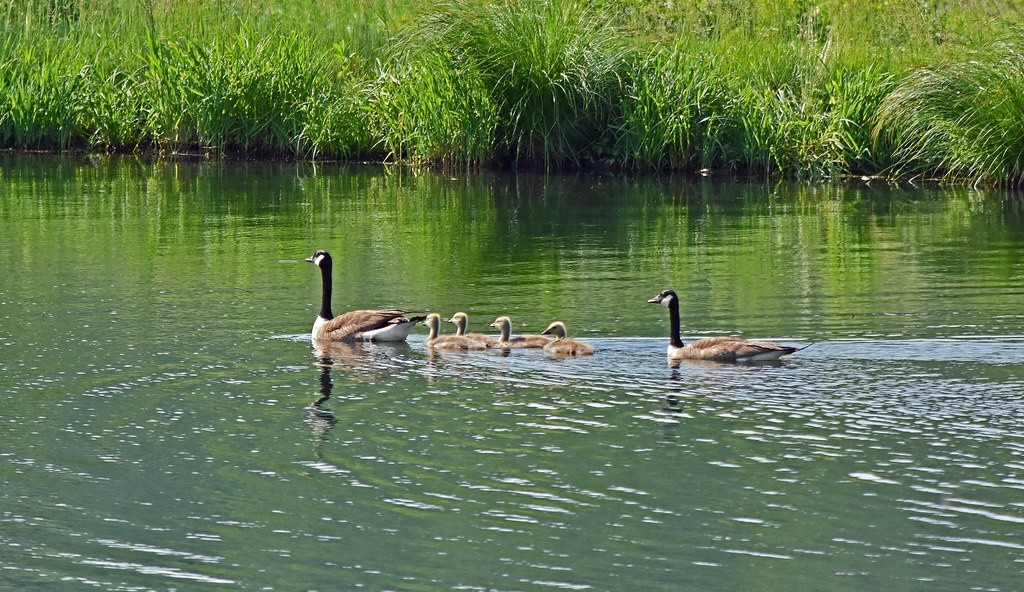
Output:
647 290 816 362
302 249 596 359
